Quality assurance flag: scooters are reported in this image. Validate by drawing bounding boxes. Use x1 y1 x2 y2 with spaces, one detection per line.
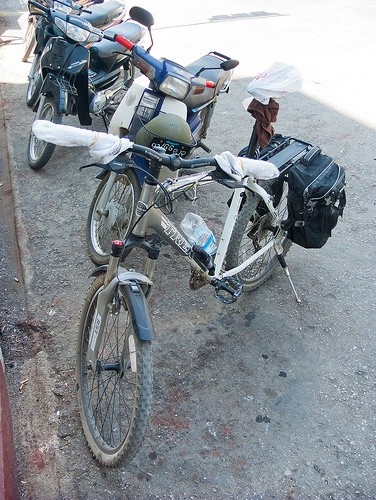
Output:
21 0 127 62
85 4 239 266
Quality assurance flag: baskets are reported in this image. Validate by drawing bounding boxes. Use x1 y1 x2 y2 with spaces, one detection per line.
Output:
131 106 196 155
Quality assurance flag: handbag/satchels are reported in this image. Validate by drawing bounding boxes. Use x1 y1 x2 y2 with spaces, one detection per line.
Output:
237 134 290 161
282 148 346 248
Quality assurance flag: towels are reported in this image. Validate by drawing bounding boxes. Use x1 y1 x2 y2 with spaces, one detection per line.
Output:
31 119 136 166
246 60 305 148
213 150 280 180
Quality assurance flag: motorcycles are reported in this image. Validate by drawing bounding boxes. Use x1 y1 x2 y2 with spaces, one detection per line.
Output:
28 0 150 170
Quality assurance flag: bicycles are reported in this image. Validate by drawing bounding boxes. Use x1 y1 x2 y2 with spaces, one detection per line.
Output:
28 63 316 468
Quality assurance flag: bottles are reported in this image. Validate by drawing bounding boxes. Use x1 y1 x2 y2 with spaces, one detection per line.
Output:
180 212 218 255
